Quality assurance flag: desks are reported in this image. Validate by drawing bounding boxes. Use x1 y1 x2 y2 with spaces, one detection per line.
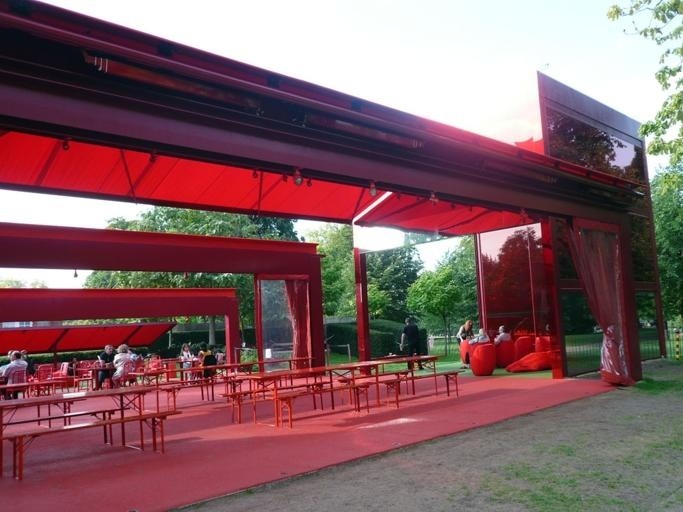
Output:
1 356 218 416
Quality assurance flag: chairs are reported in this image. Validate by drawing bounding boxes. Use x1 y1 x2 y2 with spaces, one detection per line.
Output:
459 336 549 376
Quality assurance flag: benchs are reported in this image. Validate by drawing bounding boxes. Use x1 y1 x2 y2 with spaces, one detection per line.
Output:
218 381 328 424
270 382 370 427
6 404 129 442
2 410 183 482
379 370 461 409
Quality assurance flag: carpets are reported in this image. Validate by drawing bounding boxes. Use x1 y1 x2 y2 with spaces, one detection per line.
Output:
0 370 615 511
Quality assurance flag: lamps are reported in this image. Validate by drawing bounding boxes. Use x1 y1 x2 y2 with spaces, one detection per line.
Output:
369 181 378 198
294 169 304 186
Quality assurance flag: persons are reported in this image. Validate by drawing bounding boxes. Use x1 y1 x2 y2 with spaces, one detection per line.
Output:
456 320 474 368
92 343 143 390
493 325 511 345
400 317 426 372
67 356 80 376
468 328 490 344
0 342 36 401
180 343 224 379
